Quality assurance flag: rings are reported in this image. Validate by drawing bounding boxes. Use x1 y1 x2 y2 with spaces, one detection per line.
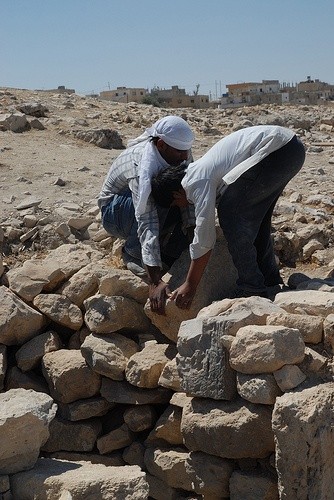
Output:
184 303 188 305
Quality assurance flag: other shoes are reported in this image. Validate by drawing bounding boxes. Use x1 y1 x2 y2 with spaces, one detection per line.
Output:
266 284 282 300
224 282 276 299
119 245 149 277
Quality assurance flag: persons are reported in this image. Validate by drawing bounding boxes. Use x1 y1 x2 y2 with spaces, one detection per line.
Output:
97 116 194 314
150 125 305 310
